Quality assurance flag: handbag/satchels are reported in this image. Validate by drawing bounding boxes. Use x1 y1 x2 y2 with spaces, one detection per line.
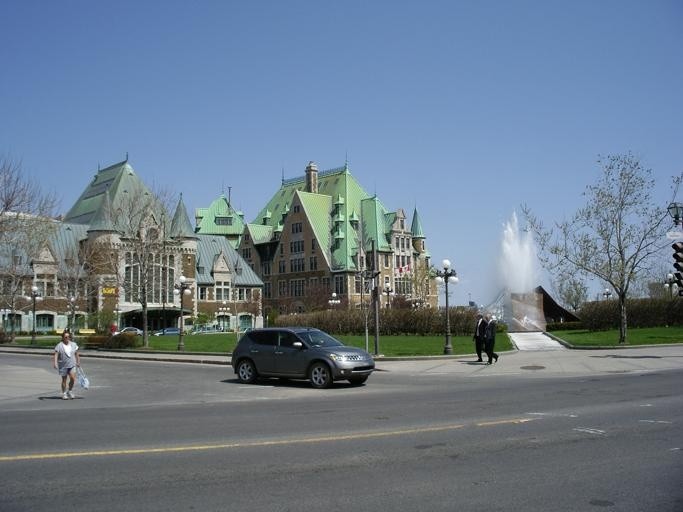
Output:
81 378 89 389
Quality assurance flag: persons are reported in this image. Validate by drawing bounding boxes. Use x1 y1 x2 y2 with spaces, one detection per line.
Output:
472 313 488 362
53 331 81 400
483 313 498 365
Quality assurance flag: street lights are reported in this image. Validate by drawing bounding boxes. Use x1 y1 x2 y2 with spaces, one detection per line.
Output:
328 292 341 320
218 301 230 332
382 283 393 309
434 260 458 354
173 275 191 351
26 286 42 345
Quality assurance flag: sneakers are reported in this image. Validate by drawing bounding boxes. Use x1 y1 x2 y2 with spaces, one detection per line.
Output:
62 390 75 400
477 354 499 366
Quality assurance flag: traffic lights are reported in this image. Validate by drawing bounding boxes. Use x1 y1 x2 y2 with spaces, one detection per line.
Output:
672 242 683 296
372 271 380 279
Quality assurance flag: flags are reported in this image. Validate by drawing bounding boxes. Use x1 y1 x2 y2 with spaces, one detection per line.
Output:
399 264 409 273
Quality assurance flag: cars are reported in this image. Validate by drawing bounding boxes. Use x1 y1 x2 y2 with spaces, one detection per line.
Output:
111 326 143 337
190 327 222 336
231 326 375 389
153 327 179 337
